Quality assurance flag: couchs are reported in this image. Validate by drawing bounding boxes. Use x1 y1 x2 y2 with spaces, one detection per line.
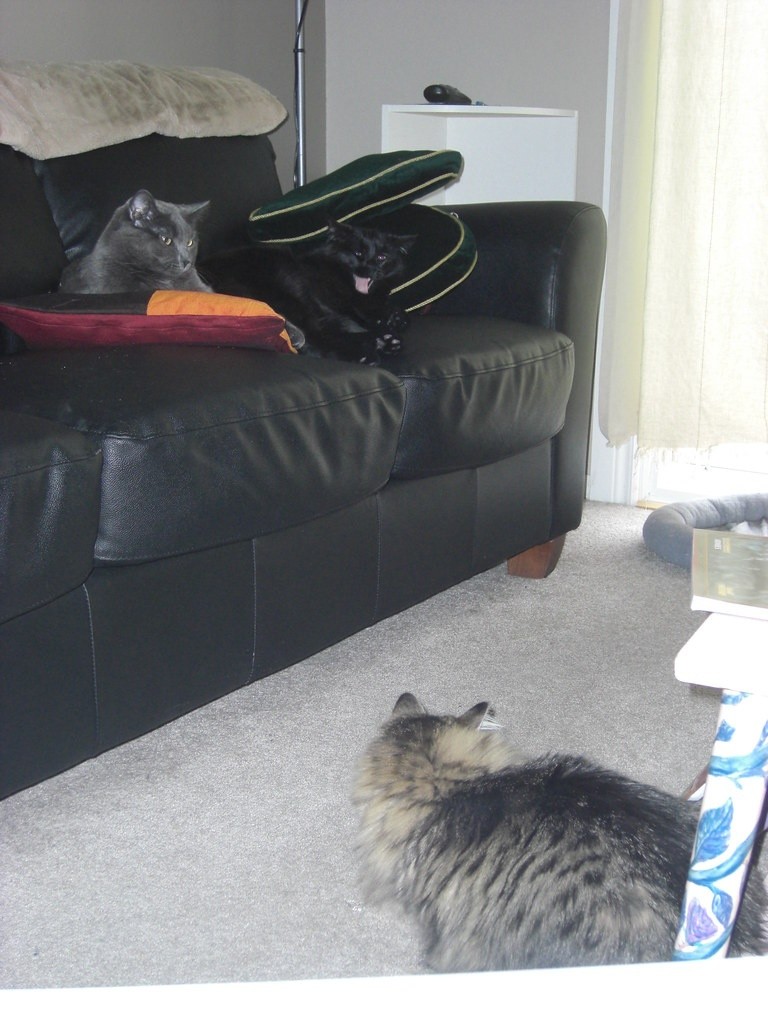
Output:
0 57 611 805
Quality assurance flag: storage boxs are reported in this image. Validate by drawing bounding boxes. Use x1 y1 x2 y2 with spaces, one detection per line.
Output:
377 102 583 208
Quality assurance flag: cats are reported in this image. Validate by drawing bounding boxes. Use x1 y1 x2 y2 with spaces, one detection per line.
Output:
352 693 767 975
55 188 215 295
194 215 419 366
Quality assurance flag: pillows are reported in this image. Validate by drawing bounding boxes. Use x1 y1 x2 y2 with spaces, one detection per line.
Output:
0 288 304 351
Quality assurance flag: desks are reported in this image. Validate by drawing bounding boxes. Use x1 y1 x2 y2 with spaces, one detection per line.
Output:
671 615 768 965
